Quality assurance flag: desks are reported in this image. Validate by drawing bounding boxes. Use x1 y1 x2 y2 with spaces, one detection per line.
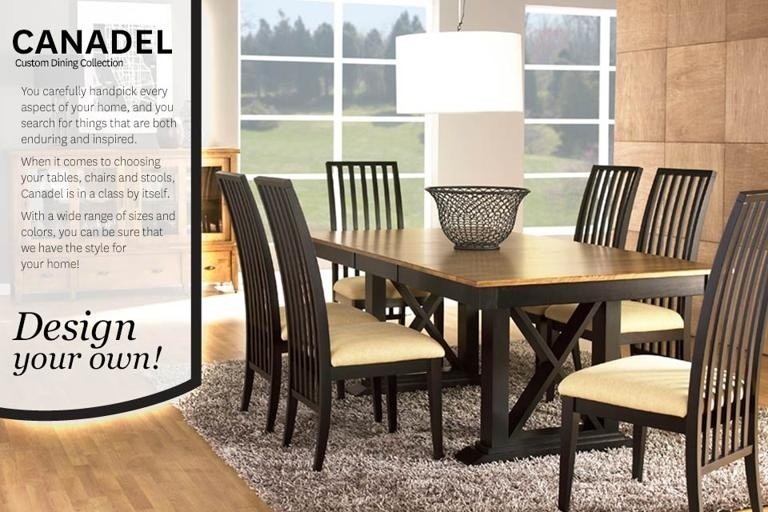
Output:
304 218 717 467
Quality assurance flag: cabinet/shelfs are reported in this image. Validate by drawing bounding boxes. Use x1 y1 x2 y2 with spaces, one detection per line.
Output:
10 147 240 301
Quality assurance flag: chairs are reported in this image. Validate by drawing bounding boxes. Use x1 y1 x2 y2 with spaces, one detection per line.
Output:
558 189 768 512
252 172 449 469
322 159 444 340
549 169 717 396
512 165 642 340
215 167 289 432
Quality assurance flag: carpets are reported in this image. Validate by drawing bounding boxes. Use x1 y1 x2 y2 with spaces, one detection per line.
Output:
171 331 768 512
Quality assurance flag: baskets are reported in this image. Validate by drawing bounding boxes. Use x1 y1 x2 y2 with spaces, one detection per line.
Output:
424 186 531 251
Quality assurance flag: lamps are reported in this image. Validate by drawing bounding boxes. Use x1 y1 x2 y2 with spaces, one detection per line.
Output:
393 0 527 118
